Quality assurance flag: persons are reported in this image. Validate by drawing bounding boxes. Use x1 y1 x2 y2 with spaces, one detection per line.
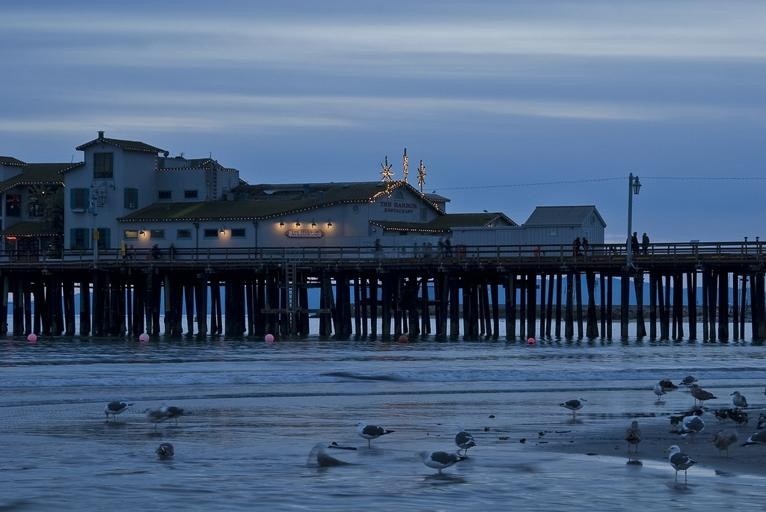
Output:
421 237 465 260
633 232 639 257
169 243 176 259
642 233 649 255
572 237 580 257
582 237 589 255
375 238 384 251
626 235 633 251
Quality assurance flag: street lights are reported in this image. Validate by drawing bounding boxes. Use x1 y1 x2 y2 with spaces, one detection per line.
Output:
626 172 641 270
